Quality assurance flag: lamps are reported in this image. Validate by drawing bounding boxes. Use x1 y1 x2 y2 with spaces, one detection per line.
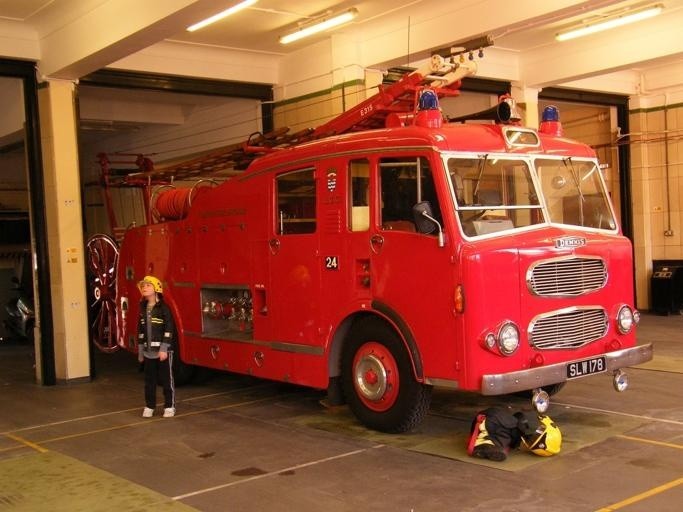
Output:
554 4 663 41
278 8 358 44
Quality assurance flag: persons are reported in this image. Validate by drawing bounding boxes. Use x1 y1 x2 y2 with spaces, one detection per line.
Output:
135 274 178 419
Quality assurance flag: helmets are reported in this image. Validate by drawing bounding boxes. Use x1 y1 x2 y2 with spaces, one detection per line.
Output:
136 276 162 294
520 413 561 458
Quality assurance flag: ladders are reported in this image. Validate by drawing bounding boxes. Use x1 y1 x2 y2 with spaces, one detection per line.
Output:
131 127 313 180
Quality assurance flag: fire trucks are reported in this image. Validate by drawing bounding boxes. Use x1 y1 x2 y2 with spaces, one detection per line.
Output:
83 54 654 434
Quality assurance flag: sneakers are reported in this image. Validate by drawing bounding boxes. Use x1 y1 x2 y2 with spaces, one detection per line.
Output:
162 407 176 418
141 405 155 418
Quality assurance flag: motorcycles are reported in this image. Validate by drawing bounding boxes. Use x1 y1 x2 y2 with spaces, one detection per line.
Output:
1 276 35 341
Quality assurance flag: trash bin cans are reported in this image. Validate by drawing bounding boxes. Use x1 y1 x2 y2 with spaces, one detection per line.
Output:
651 262 683 316
